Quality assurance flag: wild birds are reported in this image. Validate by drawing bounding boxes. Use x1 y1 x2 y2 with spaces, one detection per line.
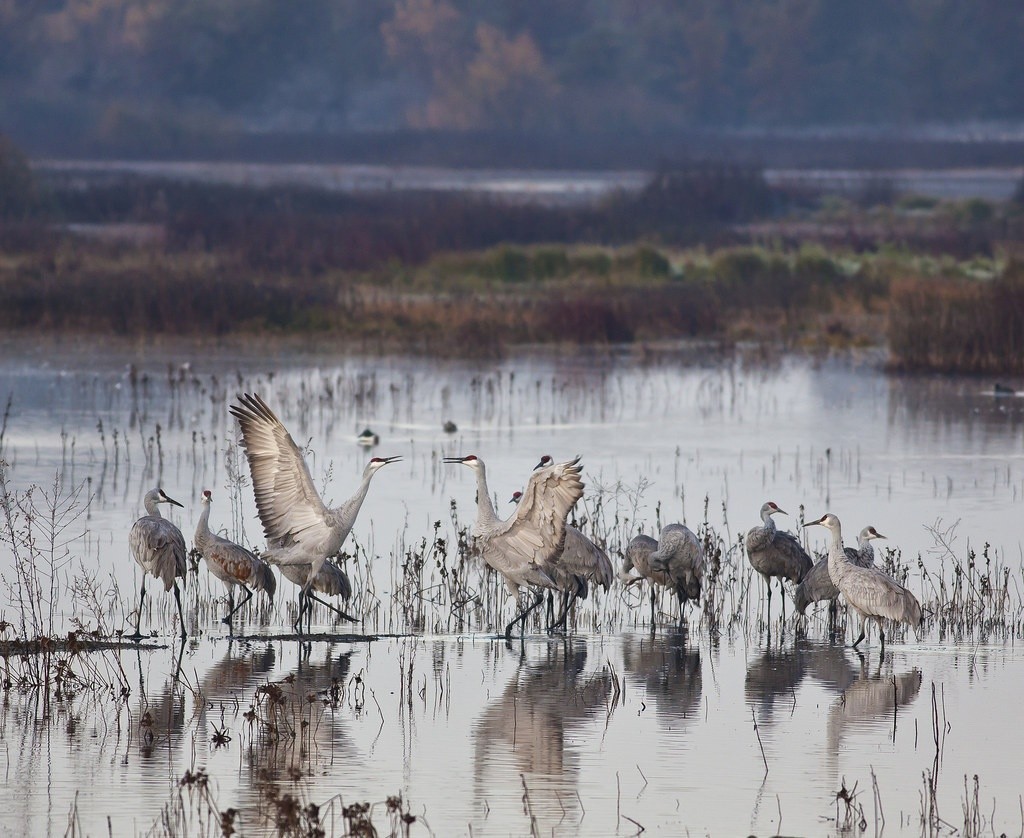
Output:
194 490 277 622
802 513 923 652
618 524 706 626
793 525 889 631
747 502 814 632
443 454 585 638
229 391 403 636
266 532 361 638
508 456 614 635
123 487 188 639
